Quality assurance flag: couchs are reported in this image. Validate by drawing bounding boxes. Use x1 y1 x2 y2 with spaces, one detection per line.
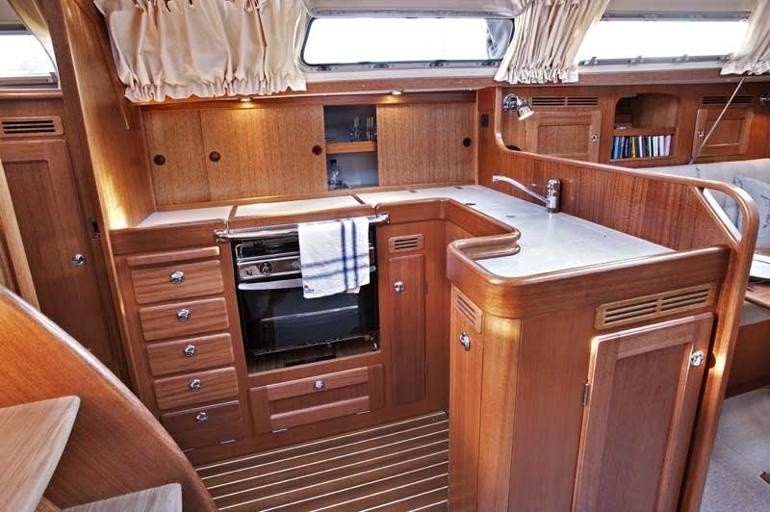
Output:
623 157 770 401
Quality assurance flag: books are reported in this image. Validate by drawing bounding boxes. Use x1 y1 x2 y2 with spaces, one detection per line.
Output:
610 132 672 159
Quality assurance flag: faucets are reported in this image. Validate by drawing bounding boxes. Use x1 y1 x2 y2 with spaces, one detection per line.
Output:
491 173 562 214
